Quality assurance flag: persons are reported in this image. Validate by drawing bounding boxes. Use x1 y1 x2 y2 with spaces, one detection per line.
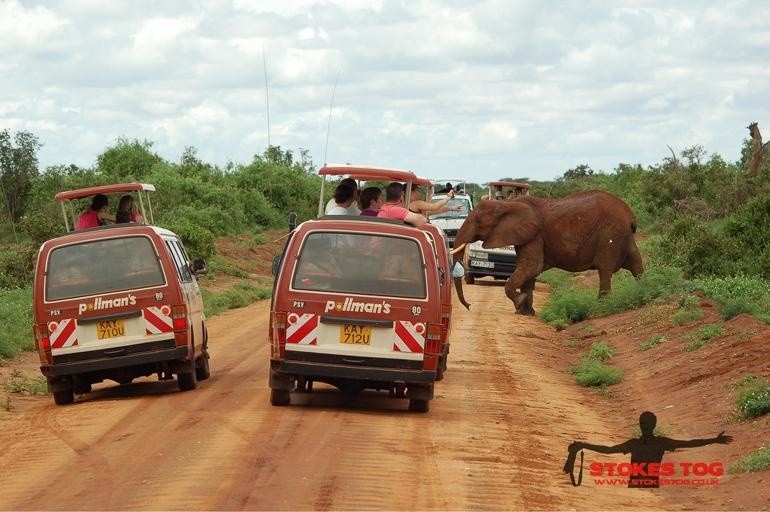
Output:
303 177 522 280
74 193 144 277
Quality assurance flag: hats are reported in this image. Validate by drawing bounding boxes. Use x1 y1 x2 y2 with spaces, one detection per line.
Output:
495 191 504 196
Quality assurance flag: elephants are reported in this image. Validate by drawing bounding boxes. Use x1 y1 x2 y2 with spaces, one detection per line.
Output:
448 190 643 316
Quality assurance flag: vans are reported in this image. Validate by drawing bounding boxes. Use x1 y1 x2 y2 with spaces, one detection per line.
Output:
267 166 444 412
30 181 210 406
462 181 530 285
412 178 454 382
426 179 474 249
